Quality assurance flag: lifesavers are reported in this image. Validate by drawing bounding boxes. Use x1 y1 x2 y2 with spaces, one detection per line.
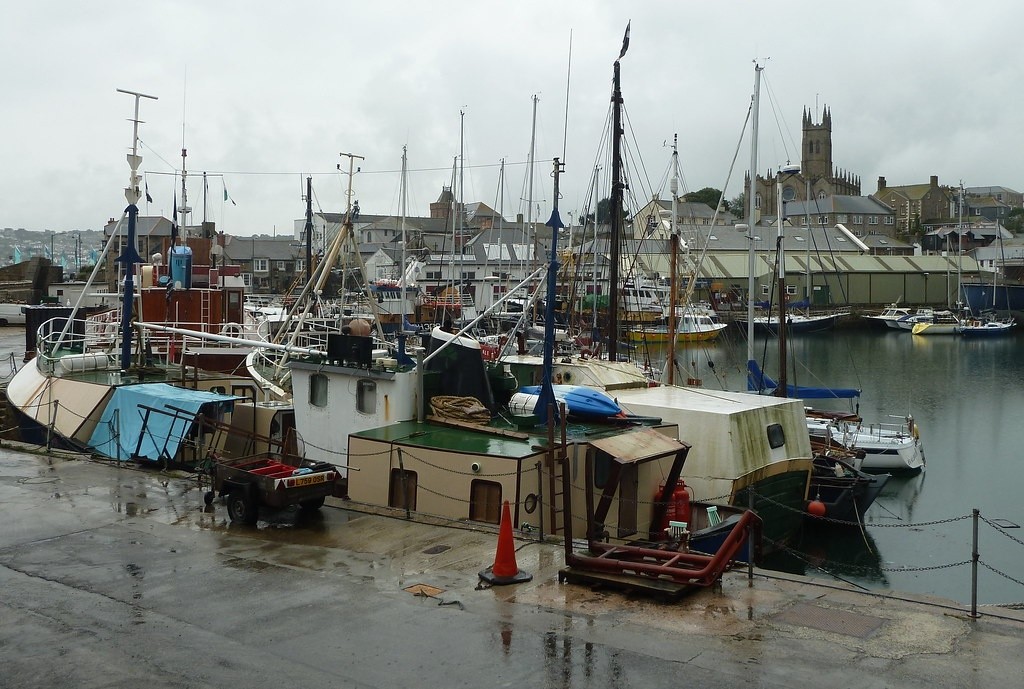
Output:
221 322 243 339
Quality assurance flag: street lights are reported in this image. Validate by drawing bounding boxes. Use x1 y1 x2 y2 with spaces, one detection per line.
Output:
72 236 77 274
79 234 82 274
52 233 68 266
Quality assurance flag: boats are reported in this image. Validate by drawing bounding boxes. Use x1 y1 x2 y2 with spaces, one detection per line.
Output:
862 178 1024 335
510 386 622 422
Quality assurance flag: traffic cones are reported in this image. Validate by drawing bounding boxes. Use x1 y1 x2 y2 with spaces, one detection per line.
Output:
478 500 533 585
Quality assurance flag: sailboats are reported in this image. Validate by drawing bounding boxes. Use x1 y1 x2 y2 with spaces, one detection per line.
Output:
8 20 927 560
